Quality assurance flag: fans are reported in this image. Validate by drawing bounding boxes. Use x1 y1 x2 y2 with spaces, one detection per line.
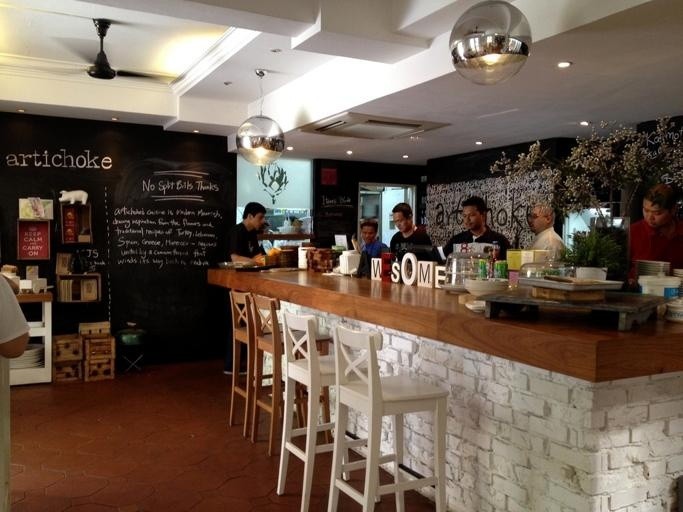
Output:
0 18 185 84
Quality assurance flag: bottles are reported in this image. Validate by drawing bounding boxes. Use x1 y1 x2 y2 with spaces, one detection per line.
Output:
491 240 501 261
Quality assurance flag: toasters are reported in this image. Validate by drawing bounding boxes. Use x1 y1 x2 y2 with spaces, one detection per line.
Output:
339 250 362 274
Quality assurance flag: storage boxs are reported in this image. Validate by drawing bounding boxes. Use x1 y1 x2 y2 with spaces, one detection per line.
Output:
52 332 117 384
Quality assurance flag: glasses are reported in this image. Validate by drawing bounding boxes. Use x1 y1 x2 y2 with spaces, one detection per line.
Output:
525 213 549 219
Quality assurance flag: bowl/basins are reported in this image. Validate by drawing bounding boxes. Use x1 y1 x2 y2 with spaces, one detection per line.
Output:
464 277 509 297
278 225 300 234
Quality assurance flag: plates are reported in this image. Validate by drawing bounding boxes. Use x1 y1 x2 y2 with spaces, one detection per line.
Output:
633 260 683 294
465 300 486 314
8 344 43 369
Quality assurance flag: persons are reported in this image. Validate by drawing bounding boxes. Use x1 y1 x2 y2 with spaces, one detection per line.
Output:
442 196 511 261
389 202 433 256
0 273 30 359
628 185 683 284
221 201 311 376
527 204 567 260
359 220 389 256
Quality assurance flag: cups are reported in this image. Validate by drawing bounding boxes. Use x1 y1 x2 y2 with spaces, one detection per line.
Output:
298 247 315 270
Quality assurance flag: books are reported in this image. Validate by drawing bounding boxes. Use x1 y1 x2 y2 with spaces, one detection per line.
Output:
61 279 73 302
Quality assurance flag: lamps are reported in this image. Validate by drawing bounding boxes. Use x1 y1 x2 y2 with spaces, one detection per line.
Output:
447 0 534 88
235 67 286 166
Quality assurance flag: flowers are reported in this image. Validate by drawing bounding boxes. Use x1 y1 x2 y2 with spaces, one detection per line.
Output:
489 111 683 230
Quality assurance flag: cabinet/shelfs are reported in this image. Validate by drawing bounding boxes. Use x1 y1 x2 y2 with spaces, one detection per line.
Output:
8 291 54 386
56 273 102 303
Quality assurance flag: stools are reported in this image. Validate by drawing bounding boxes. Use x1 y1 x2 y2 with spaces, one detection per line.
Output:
226 287 304 438
250 293 334 456
276 309 380 510
328 322 449 510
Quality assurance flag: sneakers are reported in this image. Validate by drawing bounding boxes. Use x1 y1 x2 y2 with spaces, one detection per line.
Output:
224 366 247 375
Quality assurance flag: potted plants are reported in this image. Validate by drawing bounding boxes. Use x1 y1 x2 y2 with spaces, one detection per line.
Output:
559 224 623 281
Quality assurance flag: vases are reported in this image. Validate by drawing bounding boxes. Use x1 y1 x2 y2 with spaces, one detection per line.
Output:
589 217 631 275
